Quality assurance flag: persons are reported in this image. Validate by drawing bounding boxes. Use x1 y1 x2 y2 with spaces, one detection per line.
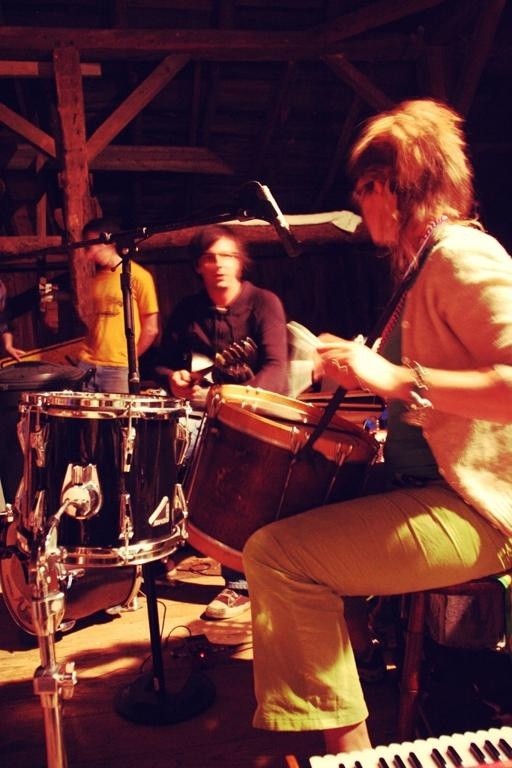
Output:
0 280 26 362
77 218 159 394
139 225 287 620
244 100 512 755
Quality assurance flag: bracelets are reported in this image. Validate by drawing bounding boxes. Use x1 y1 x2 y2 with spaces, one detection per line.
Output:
400 359 434 427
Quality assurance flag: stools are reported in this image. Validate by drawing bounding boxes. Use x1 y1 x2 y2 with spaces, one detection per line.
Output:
353 580 512 751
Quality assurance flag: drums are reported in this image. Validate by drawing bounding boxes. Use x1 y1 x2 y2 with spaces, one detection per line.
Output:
185 384 381 575
0 518 142 638
16 391 191 568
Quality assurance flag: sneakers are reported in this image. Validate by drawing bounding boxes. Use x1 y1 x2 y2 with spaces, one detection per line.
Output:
137 559 177 577
205 573 250 619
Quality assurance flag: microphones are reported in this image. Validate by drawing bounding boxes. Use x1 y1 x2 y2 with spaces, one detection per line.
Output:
253 183 303 257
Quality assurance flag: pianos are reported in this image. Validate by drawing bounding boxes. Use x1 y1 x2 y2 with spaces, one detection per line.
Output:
285 725 512 768
